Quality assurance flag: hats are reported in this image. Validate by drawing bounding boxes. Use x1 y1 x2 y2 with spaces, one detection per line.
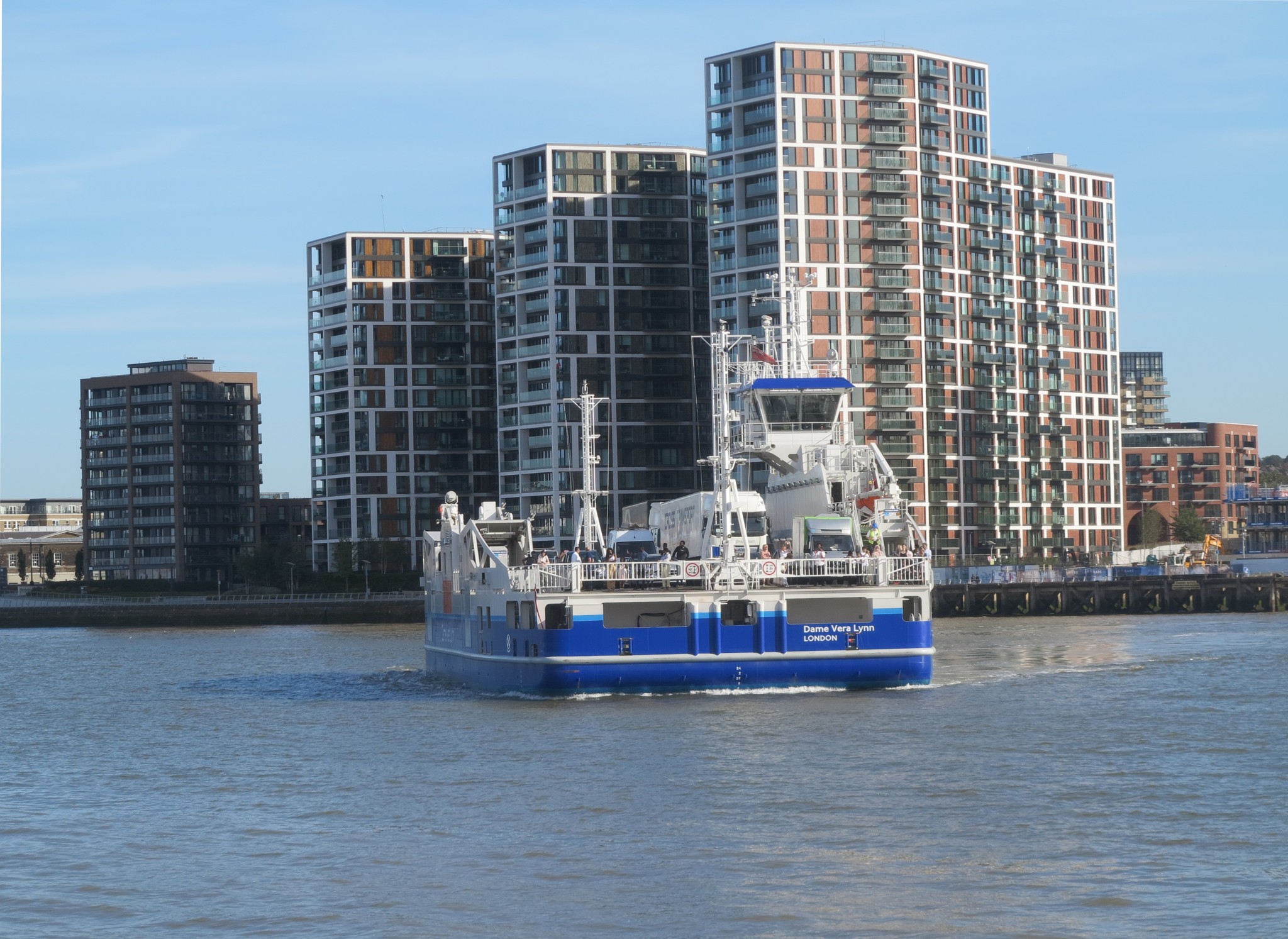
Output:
872 523 877 528
541 549 548 554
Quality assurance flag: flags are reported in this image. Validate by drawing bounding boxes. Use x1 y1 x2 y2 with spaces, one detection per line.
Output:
751 344 777 365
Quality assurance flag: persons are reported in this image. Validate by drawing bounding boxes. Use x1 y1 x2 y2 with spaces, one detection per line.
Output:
522 523 934 591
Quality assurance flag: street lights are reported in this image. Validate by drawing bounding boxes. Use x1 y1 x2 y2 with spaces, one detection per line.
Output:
1109 537 1118 565
287 561 296 601
216 569 222 601
361 560 372 598
988 540 996 559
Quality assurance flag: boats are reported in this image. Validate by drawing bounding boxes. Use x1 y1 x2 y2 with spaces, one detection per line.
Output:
421 270 939 700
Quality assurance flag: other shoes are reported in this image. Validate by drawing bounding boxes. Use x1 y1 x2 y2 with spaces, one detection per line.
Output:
763 577 926 589
580 584 685 592
